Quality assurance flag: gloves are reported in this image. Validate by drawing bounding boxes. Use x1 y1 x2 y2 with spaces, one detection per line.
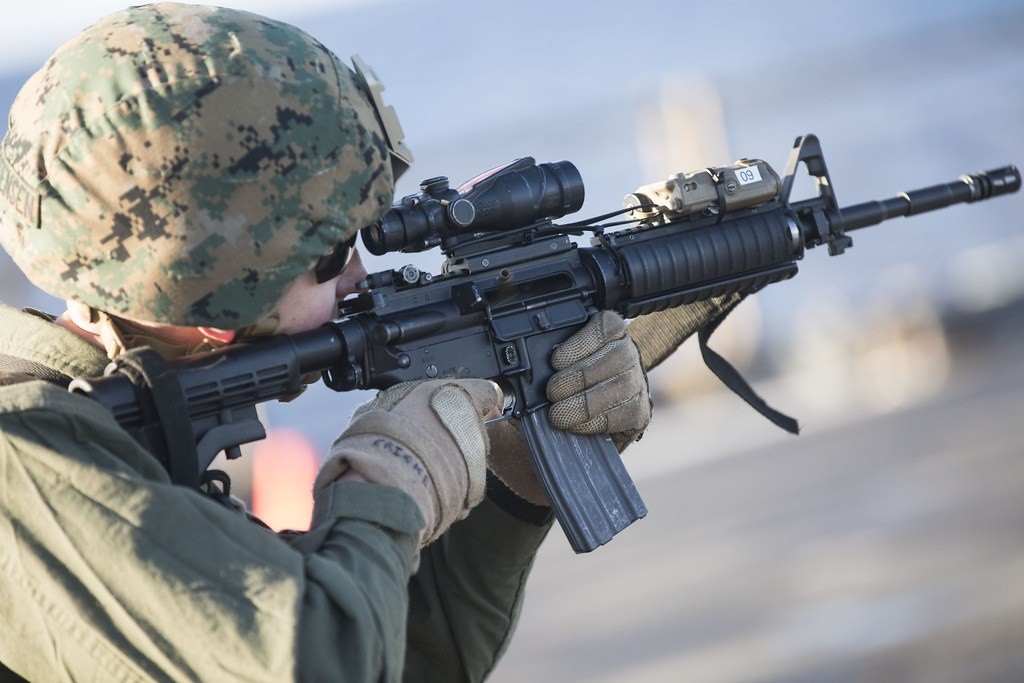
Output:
482 310 654 507
312 378 505 550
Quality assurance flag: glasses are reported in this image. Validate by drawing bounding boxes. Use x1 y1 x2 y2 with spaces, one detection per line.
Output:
314 230 357 285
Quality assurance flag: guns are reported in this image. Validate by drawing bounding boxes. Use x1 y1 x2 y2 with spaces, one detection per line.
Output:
86 130 1022 557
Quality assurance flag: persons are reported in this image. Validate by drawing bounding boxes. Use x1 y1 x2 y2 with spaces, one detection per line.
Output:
0 4 651 683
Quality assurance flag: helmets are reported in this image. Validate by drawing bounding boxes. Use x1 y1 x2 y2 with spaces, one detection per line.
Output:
0 2 414 331
